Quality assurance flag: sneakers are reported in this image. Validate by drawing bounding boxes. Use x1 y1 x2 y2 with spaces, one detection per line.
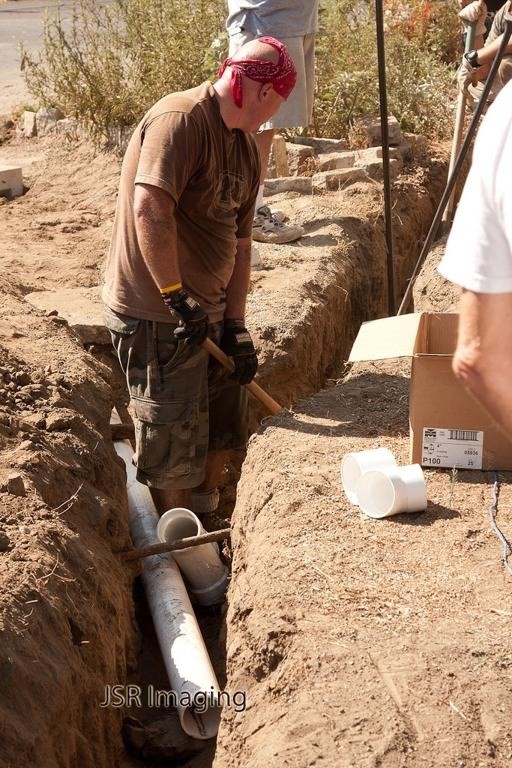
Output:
252 206 303 244
193 511 231 532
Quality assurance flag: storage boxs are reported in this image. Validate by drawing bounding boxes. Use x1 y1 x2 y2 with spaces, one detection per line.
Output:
343 314 512 472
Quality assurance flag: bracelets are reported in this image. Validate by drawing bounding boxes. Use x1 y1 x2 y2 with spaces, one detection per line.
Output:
158 279 183 295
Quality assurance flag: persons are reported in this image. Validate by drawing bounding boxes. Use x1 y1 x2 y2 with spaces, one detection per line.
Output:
435 78 512 433
451 0 512 116
221 1 323 245
100 32 298 527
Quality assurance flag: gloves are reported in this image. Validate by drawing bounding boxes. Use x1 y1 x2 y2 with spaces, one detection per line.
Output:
457 53 477 101
209 319 258 385
161 287 209 345
456 0 487 38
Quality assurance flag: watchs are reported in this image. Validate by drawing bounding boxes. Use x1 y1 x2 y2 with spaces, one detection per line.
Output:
466 48 482 70
